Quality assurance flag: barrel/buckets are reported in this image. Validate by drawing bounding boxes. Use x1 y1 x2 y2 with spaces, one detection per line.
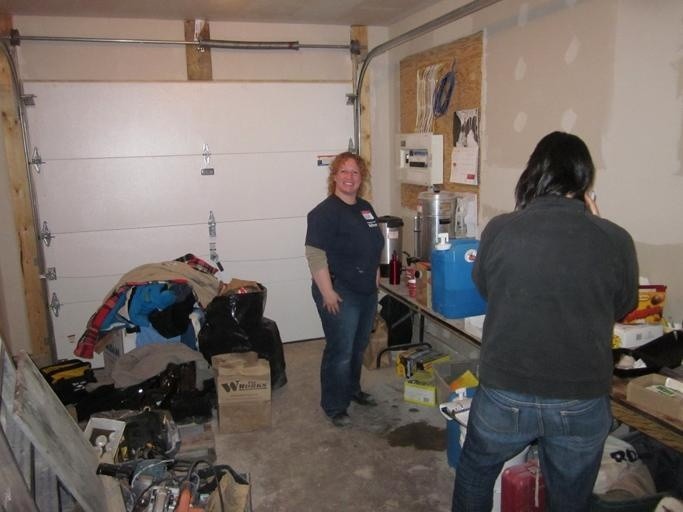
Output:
440 387 546 511
431 233 488 319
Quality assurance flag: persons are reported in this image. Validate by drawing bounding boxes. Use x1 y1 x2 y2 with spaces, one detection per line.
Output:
451 131 639 512
304 153 385 429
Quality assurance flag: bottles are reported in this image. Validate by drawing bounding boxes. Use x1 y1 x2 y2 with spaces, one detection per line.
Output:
390 254 401 285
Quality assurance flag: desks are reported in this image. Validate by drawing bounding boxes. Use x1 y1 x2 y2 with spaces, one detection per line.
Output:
375 268 681 458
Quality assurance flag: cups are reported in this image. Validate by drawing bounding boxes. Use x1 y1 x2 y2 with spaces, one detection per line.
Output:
408 279 416 297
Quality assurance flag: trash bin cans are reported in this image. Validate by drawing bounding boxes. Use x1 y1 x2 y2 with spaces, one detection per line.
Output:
380 296 413 349
587 455 682 512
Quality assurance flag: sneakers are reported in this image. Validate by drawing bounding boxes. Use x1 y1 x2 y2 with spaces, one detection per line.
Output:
332 391 374 429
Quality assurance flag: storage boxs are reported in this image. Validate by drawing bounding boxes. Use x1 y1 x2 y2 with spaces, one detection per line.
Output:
430 357 480 471
212 349 270 432
